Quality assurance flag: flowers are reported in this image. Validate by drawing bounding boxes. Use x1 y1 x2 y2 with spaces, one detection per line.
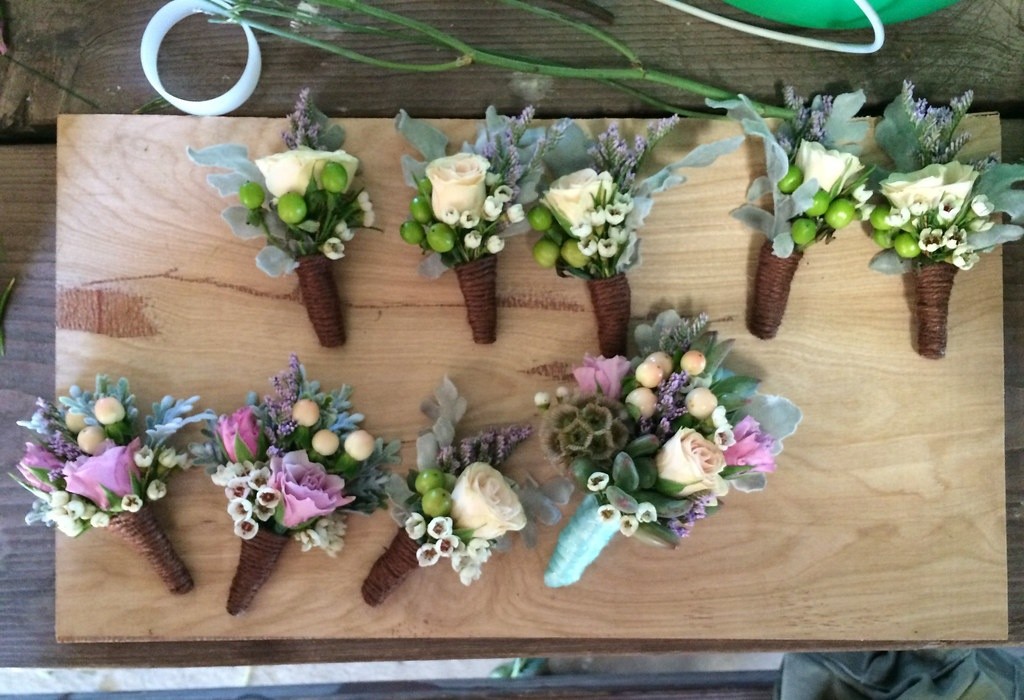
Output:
5 77 1024 616
